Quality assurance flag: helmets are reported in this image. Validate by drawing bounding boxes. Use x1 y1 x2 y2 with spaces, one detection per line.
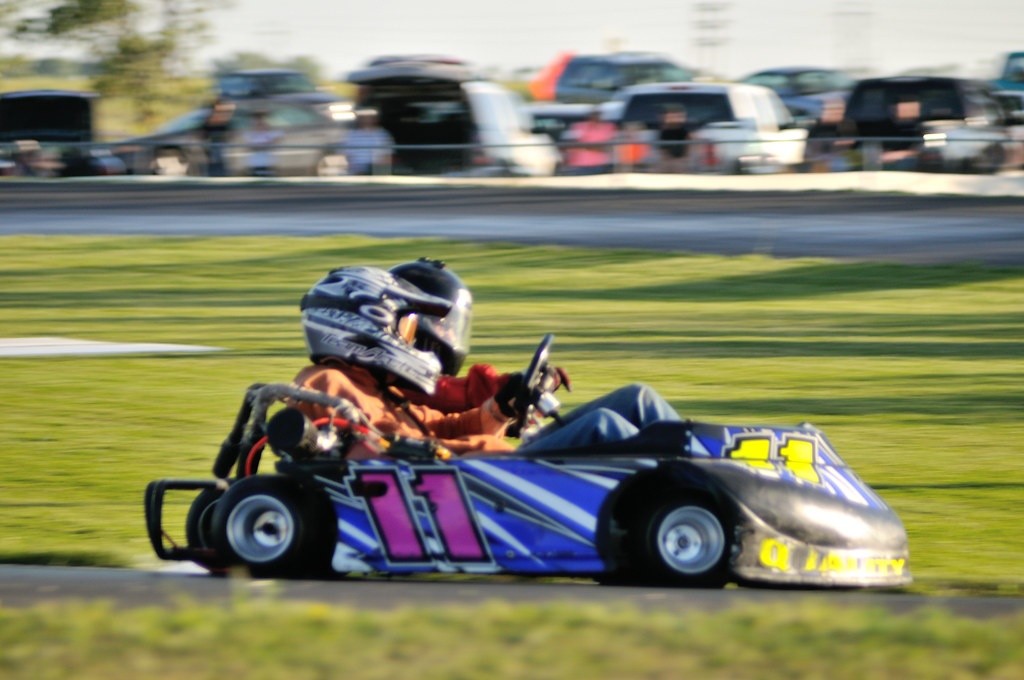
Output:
302 257 473 394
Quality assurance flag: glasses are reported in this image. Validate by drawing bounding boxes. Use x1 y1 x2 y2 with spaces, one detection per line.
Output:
436 314 451 328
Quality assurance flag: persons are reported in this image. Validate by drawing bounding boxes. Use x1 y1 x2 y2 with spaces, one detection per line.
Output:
327 104 396 176
287 258 571 466
564 105 697 175
184 99 280 177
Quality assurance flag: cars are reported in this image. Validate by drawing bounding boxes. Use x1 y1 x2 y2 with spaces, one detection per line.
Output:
0 50 1024 178
